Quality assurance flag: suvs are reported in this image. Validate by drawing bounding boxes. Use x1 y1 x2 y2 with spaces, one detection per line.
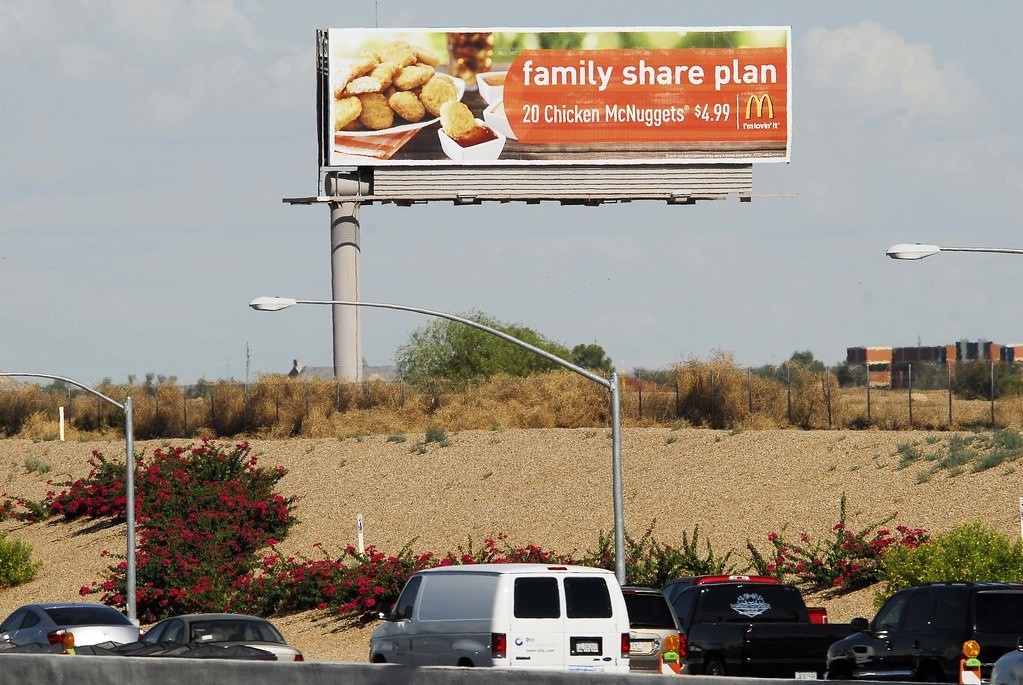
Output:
824 580 1023 685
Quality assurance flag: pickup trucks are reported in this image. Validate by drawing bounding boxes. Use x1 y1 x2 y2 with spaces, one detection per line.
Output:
658 575 857 679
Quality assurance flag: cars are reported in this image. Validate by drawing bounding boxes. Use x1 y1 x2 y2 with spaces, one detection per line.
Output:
619 586 687 674
136 613 304 662
0 601 143 650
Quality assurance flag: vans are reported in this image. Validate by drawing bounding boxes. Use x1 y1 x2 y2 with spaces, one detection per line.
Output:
365 561 631 674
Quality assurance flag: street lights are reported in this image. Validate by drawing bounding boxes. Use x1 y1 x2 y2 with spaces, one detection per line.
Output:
248 295 633 588
